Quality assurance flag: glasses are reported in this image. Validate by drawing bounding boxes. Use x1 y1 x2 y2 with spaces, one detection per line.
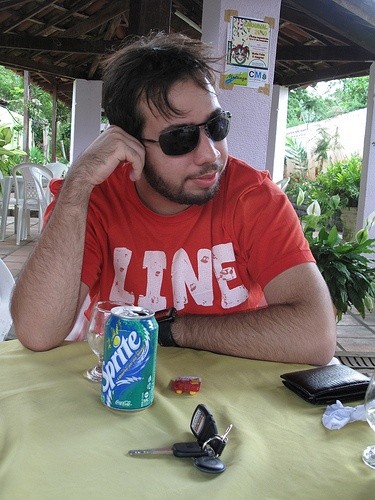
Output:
139 110 232 156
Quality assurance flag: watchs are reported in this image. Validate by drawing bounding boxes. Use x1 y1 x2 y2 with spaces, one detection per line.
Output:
154 307 181 347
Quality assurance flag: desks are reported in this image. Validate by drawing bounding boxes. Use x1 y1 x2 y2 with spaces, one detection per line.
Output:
1 339 375 500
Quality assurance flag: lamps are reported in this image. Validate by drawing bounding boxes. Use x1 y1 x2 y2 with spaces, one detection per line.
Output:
172 8 201 34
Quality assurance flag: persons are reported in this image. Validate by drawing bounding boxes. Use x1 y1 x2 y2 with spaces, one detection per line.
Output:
11 34 336 367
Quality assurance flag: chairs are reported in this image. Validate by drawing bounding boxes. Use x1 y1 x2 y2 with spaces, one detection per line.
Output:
0 162 68 246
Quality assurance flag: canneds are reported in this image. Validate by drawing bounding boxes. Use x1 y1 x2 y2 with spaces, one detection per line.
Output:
100 306 158 411
